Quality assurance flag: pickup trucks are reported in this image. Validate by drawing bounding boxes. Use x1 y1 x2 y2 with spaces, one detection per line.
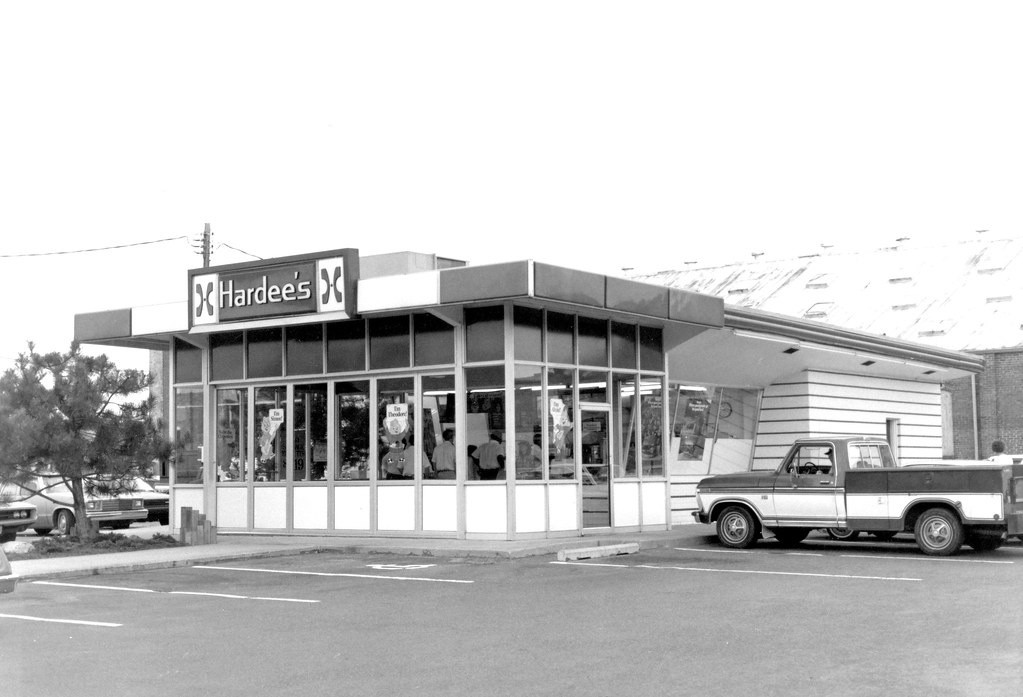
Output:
690 436 1023 556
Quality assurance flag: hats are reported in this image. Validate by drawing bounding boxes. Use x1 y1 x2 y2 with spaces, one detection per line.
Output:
824 448 832 455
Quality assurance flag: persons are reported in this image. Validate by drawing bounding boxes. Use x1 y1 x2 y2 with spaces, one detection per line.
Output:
380 431 557 480
988 441 1013 464
815 447 834 475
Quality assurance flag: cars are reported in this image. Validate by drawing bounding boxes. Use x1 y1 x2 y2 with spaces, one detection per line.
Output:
1 471 148 536
0 499 39 544
826 460 1022 541
86 474 169 526
